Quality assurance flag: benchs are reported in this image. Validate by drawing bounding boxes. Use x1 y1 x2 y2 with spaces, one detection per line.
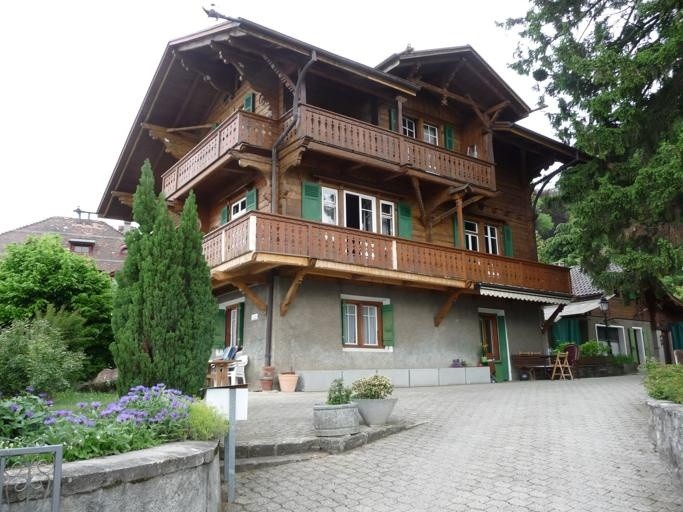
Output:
513 354 553 380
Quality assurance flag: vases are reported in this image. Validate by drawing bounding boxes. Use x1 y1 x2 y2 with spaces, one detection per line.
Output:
278 374 299 392
261 380 272 391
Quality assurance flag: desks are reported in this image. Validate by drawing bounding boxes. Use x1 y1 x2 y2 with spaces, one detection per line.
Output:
207 360 242 387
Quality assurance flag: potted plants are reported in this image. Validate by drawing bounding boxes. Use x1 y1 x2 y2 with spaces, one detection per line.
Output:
351 375 397 426
313 379 360 437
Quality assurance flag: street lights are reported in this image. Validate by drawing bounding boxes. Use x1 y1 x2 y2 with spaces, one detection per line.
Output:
597 295 614 357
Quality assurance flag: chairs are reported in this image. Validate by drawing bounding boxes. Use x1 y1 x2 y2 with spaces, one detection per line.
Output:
227 355 248 386
564 344 580 379
551 350 573 380
